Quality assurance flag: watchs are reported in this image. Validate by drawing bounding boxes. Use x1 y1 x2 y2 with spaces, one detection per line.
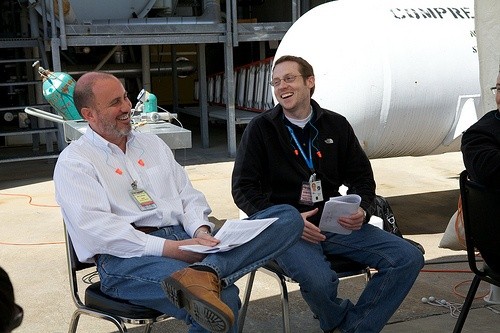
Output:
359 207 370 223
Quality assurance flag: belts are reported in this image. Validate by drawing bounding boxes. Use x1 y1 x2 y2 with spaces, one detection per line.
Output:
93 225 157 267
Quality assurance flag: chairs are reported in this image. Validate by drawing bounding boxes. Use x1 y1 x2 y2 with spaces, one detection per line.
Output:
452 171 500 333
64 220 241 333
238 197 424 333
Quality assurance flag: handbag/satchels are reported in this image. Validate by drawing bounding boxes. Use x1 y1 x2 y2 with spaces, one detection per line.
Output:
440 195 480 252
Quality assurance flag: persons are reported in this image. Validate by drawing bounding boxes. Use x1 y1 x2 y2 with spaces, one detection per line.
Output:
459 62 500 292
231 56 425 333
54 72 303 333
312 183 316 192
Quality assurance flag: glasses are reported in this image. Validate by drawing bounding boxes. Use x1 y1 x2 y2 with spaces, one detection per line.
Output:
491 86 500 95
270 73 304 86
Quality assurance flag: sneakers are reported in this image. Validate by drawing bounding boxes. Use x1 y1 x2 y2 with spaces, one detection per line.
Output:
162 268 235 333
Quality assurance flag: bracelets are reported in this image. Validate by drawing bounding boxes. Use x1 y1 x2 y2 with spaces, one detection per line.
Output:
195 228 209 239
